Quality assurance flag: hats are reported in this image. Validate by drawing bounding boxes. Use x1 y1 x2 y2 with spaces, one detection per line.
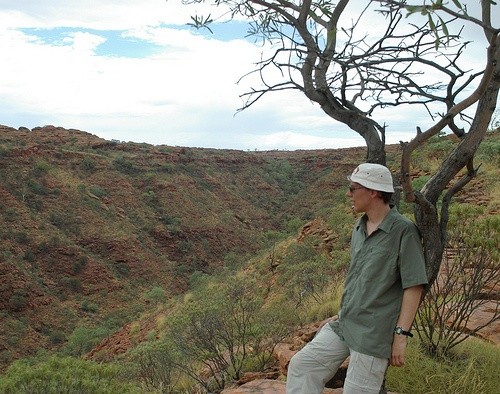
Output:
347 163 395 193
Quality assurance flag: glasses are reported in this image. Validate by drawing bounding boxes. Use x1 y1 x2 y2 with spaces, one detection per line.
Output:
349 184 364 192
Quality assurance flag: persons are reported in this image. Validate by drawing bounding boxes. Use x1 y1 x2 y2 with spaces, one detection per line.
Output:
285 162 429 394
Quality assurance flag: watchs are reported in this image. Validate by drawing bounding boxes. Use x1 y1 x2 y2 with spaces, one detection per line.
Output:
394 326 413 337
